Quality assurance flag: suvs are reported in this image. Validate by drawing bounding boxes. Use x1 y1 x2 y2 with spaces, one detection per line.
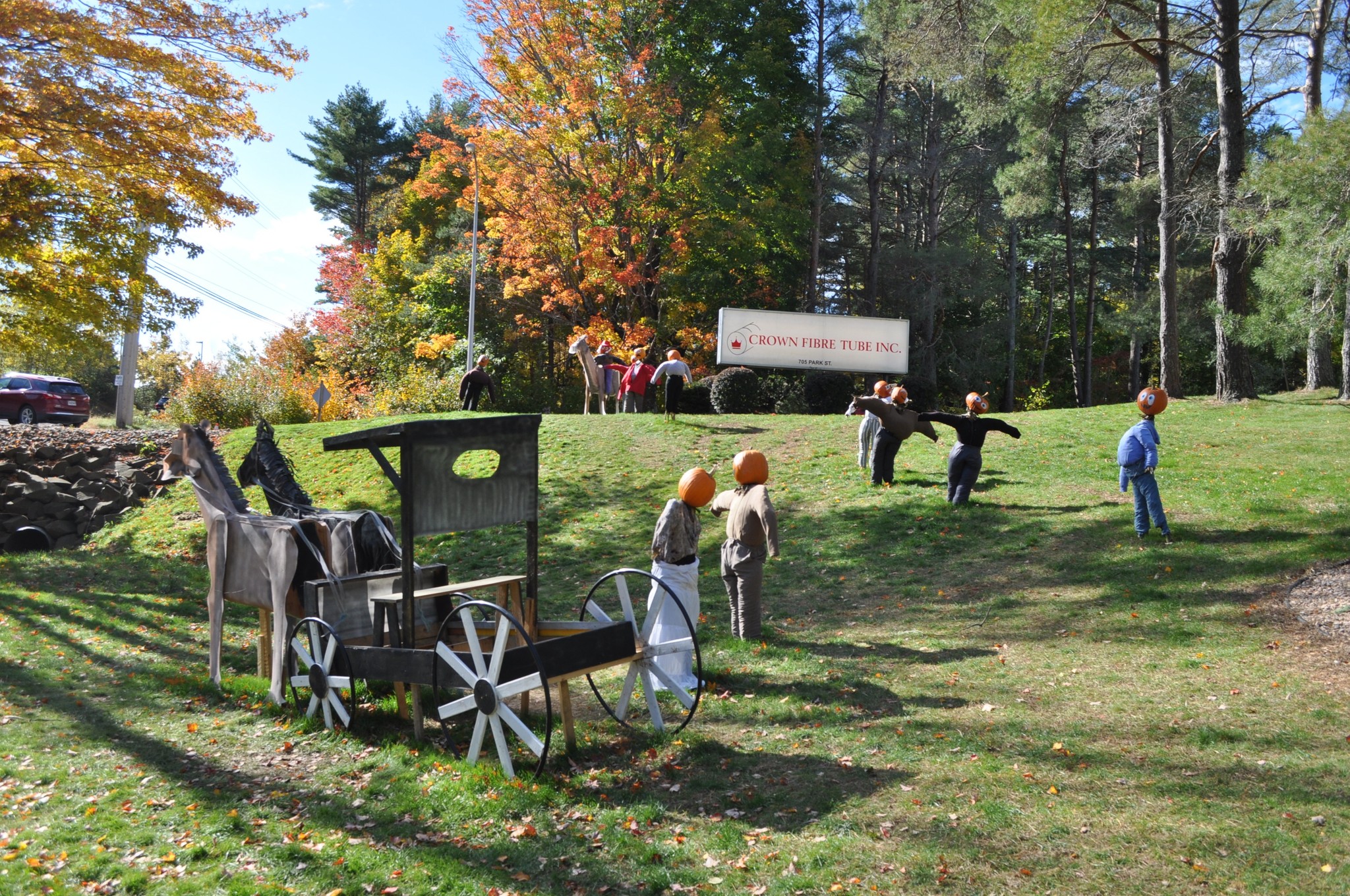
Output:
154 394 171 414
0 372 91 428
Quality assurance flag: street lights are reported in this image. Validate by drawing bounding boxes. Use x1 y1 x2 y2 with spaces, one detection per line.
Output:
196 341 203 362
461 142 481 408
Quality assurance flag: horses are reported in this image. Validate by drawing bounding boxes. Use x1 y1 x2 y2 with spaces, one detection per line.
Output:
159 417 403 707
569 335 623 415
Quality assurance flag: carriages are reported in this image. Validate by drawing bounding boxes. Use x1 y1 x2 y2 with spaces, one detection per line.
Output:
152 406 705 788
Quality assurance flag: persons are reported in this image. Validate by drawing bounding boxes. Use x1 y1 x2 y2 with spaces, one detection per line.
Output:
1117 388 1173 546
642 461 719 693
591 335 693 424
846 379 1021 509
710 445 778 640
459 352 495 411
